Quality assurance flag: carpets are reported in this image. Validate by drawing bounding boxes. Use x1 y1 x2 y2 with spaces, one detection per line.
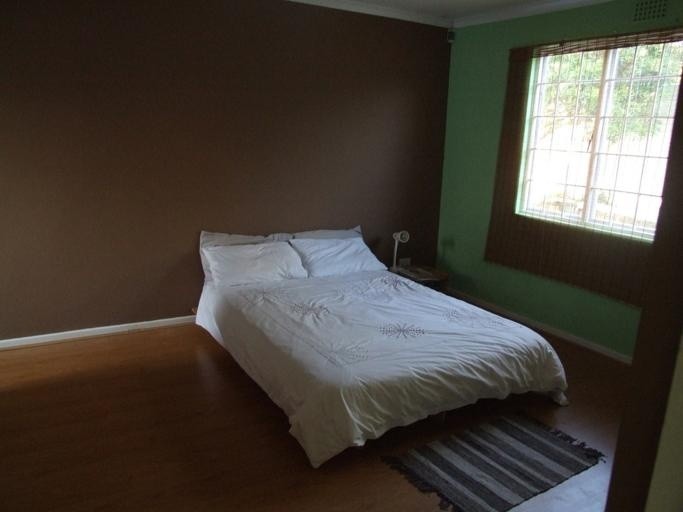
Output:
378 398 604 511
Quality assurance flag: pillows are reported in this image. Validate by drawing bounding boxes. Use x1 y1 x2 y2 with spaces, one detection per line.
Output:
292 229 386 281
293 224 362 238
201 240 307 285
199 228 293 246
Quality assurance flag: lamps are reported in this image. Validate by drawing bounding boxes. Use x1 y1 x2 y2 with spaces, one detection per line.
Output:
390 230 410 275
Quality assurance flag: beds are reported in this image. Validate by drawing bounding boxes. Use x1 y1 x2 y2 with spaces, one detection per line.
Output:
195 216 551 447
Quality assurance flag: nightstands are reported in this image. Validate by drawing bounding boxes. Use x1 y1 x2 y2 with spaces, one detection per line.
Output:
401 266 453 296
